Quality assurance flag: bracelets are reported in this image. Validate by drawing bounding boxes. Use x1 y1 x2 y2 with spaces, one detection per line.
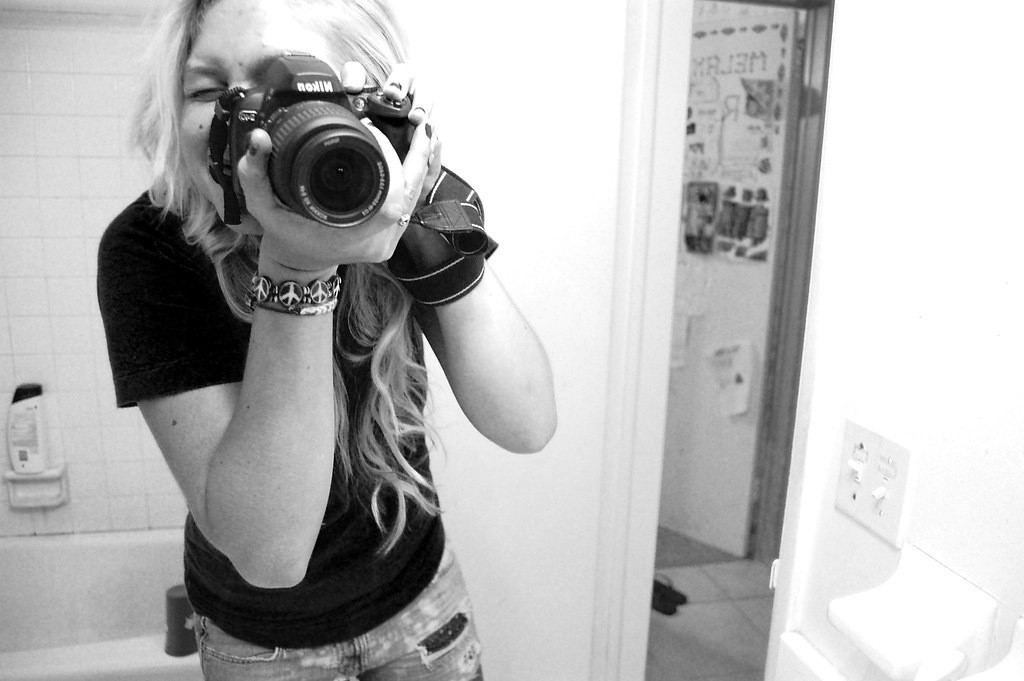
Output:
246 275 342 316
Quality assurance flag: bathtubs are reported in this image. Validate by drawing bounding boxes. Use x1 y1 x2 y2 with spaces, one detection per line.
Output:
0 527 205 681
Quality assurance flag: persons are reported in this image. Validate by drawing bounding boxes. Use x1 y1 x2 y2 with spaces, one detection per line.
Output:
97 0 557 681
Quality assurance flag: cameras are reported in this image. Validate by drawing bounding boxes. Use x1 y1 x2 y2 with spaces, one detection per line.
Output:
206 54 415 227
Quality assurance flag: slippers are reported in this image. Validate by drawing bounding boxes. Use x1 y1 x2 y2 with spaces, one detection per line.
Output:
651 573 687 616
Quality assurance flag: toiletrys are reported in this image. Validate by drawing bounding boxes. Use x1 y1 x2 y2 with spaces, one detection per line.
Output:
7 384 46 475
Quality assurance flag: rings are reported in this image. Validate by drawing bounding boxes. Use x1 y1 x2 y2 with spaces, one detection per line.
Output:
398 214 410 227
